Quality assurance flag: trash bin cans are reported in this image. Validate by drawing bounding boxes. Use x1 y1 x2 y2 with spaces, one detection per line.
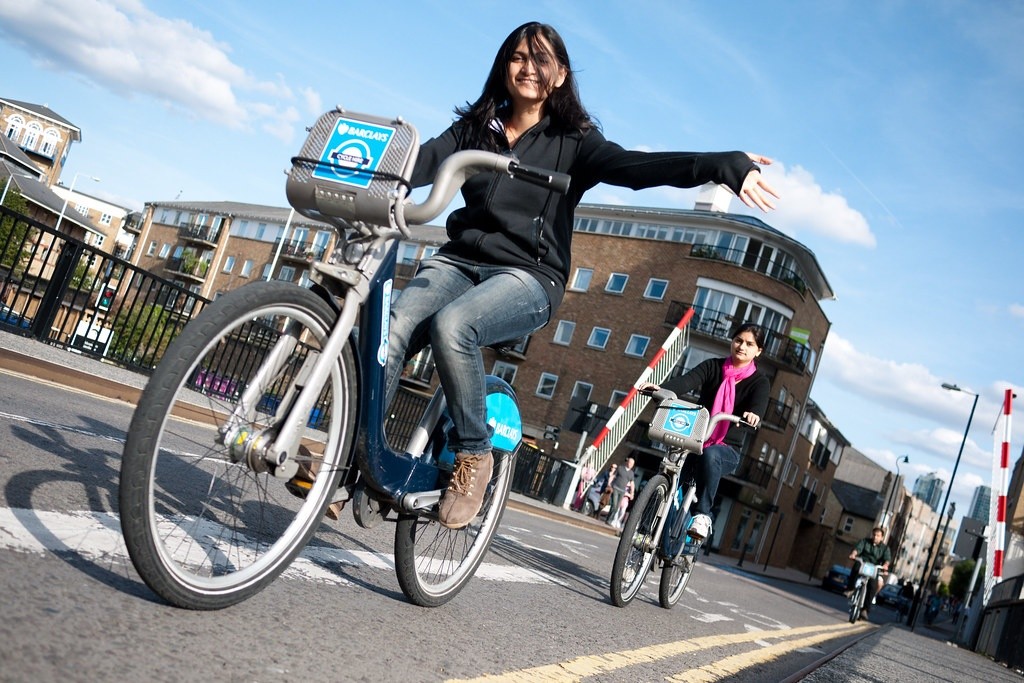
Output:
540 457 576 506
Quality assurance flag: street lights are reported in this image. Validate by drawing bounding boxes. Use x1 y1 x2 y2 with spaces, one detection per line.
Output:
907 382 980 625
880 452 909 529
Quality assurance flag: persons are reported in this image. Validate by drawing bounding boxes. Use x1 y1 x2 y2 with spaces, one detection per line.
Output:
283 20 782 528
594 456 637 528
844 527 916 625
638 323 772 539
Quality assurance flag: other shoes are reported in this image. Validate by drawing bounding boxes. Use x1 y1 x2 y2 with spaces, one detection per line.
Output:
844 590 854 598
861 610 868 619
872 596 876 604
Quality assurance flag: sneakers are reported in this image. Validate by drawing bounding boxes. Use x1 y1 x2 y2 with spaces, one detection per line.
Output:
635 534 652 548
687 513 712 540
298 445 343 520
438 451 495 528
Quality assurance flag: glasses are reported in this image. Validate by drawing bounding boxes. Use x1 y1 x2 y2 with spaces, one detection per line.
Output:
611 466 617 469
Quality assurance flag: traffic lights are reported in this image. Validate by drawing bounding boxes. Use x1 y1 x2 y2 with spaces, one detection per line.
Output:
99 287 116 308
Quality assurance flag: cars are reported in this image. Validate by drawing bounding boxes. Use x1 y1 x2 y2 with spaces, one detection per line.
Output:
560 460 601 515
0 309 30 328
823 564 851 593
876 584 902 607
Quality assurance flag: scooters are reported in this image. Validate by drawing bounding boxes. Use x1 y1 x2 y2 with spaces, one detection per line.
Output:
923 603 939 628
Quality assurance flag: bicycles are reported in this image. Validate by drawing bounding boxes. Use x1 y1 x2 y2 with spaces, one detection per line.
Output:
848 555 887 624
119 104 573 611
895 595 912 623
609 389 761 610
